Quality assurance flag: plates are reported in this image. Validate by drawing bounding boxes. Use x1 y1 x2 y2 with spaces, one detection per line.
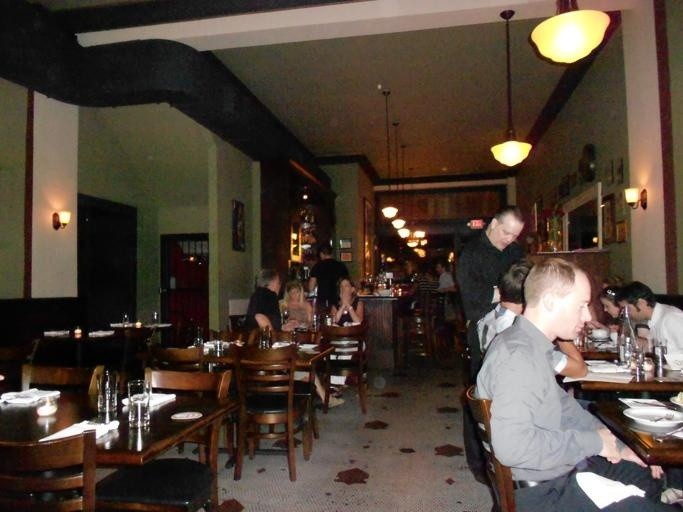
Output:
662 365 682 371
622 407 682 427
626 422 681 434
669 395 682 406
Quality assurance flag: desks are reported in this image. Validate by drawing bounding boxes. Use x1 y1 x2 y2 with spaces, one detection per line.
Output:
556 327 683 511
5 379 236 507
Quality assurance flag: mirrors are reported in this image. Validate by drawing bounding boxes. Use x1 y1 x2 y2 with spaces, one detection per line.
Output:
563 181 603 250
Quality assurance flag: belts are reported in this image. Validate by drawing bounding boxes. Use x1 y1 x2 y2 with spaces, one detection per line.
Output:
512 479 550 490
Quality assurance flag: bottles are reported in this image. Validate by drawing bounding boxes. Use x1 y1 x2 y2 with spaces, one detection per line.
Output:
192 325 204 350
619 306 667 382
259 326 273 350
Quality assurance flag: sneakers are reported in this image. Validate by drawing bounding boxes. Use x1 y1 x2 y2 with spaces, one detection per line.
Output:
328 396 345 408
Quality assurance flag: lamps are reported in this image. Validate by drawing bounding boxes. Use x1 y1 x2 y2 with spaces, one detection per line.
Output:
487 9 534 168
624 188 647 211
52 211 71 230
529 0 610 64
380 90 428 249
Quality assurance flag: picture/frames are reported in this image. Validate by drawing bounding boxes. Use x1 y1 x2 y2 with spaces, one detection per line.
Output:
231 198 246 252
339 252 353 262
601 193 616 245
616 219 627 242
339 238 352 249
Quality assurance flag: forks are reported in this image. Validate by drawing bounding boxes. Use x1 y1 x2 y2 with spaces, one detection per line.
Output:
650 412 675 426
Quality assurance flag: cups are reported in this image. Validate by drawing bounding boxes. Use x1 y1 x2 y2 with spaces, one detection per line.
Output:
127 428 152 455
37 397 58 417
123 317 129 328
94 371 155 426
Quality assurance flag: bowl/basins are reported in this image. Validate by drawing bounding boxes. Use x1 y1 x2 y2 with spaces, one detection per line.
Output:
591 328 609 340
663 354 682 368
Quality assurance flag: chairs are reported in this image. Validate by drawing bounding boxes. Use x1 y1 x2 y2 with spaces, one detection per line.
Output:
463 383 521 512
1 429 97 511
94 366 231 512
2 314 369 437
228 345 303 481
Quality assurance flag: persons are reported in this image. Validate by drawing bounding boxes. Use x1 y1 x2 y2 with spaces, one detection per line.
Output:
477 260 588 378
330 275 365 325
245 267 346 408
277 281 314 325
591 285 650 350
407 258 456 320
309 243 348 315
476 258 682 512
456 203 527 484
615 281 682 407
